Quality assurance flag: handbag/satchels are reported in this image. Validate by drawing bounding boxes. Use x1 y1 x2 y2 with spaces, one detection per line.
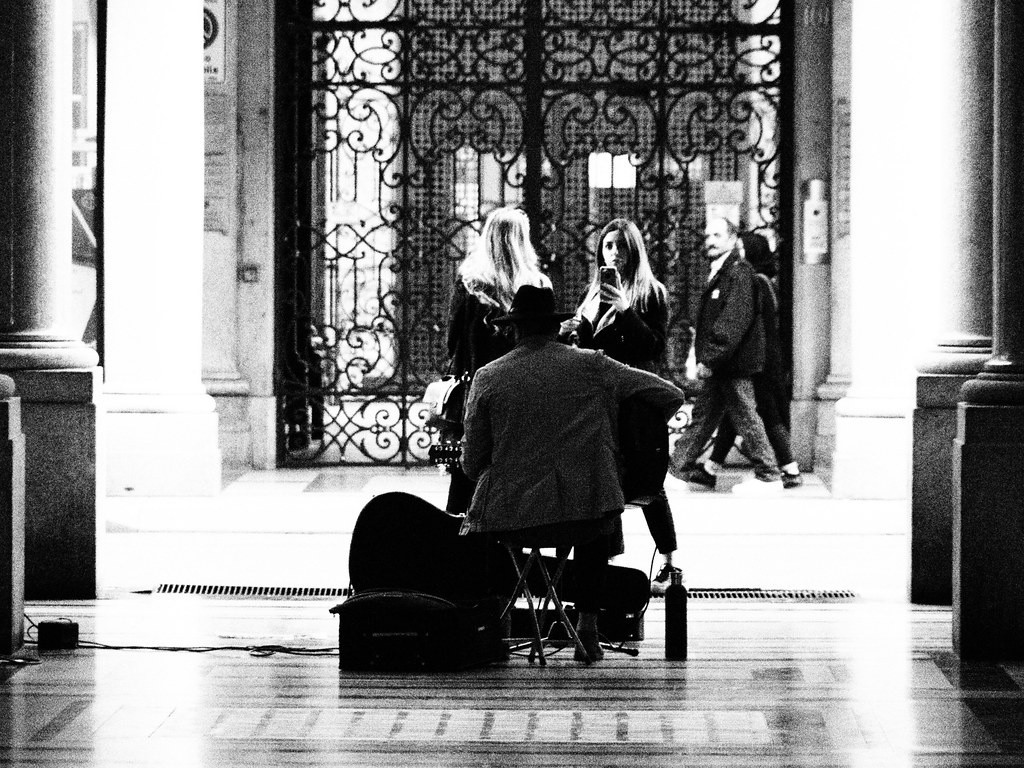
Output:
422 375 468 428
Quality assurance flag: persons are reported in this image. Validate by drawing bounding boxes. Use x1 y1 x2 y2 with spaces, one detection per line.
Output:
556 218 683 597
446 205 554 517
458 284 684 661
667 216 802 488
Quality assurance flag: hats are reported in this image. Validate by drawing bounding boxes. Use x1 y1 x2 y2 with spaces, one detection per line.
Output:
490 285 576 326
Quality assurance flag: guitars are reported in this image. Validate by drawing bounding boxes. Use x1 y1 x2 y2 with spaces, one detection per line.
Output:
429 402 670 508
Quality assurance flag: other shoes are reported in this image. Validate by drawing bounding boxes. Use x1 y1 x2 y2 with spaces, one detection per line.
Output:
667 463 717 489
781 470 802 488
574 631 604 661
756 466 781 481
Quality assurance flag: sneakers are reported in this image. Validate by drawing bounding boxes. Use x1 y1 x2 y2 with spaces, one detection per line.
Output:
651 563 686 594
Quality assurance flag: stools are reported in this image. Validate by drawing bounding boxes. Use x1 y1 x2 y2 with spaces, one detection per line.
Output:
497 536 591 668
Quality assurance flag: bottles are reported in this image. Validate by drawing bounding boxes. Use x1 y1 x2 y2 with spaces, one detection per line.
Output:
664 572 688 660
